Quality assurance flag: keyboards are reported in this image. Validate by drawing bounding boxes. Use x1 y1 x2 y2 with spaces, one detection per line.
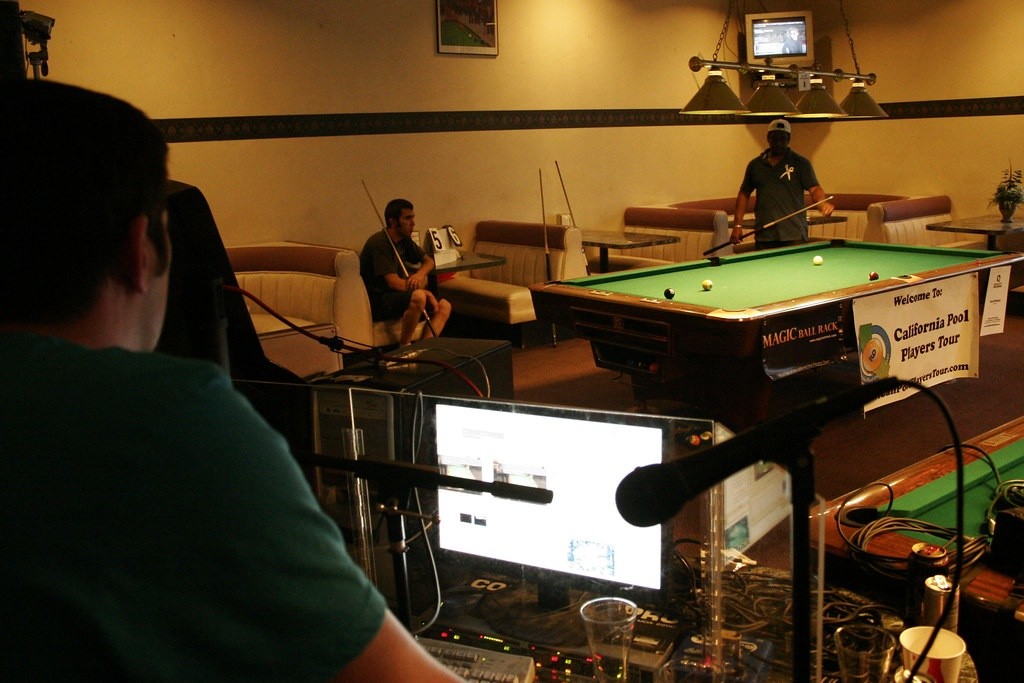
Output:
413 635 535 683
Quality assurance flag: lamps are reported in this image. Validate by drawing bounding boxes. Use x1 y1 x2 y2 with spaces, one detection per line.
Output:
680 1 888 119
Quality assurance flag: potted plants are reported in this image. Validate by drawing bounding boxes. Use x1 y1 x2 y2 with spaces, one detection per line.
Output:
987 159 1024 223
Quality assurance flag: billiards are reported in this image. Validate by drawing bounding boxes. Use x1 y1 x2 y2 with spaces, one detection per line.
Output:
868 272 879 281
702 280 713 290
664 288 675 300
813 255 823 265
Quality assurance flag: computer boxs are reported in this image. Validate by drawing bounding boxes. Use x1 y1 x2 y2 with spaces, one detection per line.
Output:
312 336 513 551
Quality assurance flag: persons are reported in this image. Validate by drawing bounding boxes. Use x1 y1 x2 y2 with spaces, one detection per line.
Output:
782 28 802 53
360 199 451 347
1 79 465 683
730 120 835 250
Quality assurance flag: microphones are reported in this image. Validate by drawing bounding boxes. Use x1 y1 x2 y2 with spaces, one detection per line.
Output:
616 372 900 527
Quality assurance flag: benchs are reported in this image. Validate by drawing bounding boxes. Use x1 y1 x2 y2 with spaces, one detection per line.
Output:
218 192 995 381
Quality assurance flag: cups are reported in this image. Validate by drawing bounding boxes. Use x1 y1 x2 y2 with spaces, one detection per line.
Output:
899 626 965 683
835 625 895 683
580 597 638 683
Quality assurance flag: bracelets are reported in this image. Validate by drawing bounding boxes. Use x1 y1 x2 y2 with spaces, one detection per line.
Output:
733 225 742 227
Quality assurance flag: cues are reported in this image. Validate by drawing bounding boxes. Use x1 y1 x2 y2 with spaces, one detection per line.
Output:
703 195 835 256
360 177 439 337
554 160 593 277
539 168 557 348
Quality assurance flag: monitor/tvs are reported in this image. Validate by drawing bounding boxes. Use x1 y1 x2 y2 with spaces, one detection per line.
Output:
426 398 674 648
744 10 815 68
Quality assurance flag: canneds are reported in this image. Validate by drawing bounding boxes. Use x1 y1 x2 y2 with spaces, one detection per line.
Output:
904 542 949 627
924 574 960 634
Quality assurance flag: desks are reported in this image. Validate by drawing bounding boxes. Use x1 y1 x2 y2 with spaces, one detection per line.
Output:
812 415 1024 671
726 216 846 229
525 236 1024 429
926 214 1024 251
581 229 681 273
405 249 507 297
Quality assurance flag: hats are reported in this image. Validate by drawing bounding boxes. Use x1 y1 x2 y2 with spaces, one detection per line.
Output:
768 119 791 133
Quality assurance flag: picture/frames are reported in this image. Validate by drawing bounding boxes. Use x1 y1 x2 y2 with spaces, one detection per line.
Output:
435 0 499 56
